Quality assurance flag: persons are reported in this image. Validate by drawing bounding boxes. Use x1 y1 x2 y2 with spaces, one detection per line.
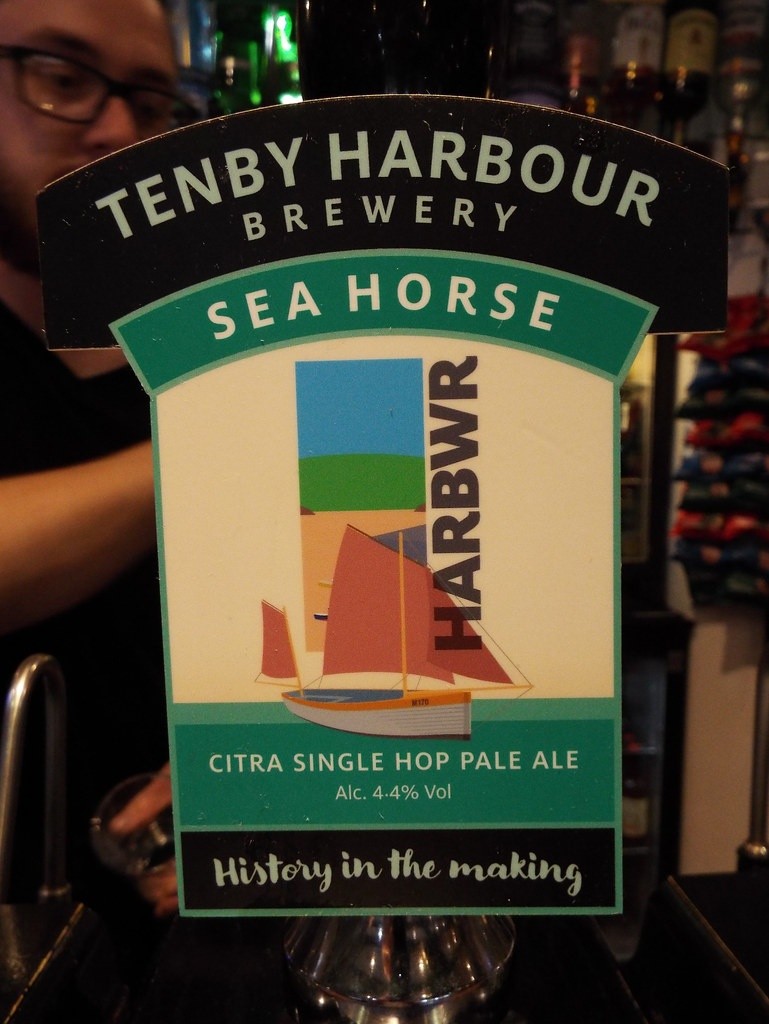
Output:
0 1 201 911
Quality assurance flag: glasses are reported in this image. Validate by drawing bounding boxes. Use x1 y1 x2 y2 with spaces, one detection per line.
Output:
0 44 203 142
